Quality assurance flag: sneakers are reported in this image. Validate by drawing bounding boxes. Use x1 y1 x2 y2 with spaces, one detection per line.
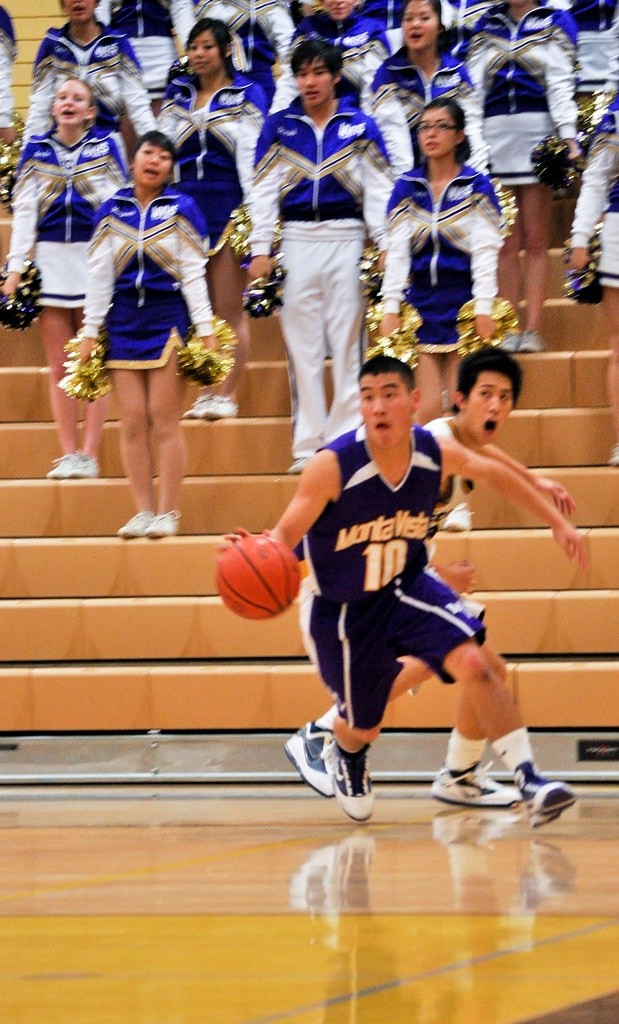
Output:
281 722 337 797
143 510 184 537
501 332 521 352
294 844 336 915
327 834 375 921
515 763 578 829
116 510 156 537
520 839 576 905
321 736 375 820
443 504 474 533
68 456 101 478
203 394 238 419
431 805 524 850
431 761 523 807
288 455 310 475
46 454 79 478
519 330 545 352
182 394 216 417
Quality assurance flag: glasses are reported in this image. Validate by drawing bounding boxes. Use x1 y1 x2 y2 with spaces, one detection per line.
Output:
415 121 458 136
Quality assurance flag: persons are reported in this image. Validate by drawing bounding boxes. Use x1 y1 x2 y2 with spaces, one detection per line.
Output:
474 1 581 351
380 98 500 427
249 39 398 472
217 354 587 828
0 79 129 478
569 63 619 465
284 347 576 810
0 1 619 354
71 130 218 538
152 19 265 418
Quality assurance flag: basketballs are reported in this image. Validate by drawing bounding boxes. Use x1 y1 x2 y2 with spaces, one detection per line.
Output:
214 535 302 620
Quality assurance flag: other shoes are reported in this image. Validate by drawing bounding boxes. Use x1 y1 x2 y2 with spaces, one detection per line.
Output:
608 443 619 466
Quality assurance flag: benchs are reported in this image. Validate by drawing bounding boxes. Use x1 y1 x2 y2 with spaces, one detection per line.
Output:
0 1 618 731
